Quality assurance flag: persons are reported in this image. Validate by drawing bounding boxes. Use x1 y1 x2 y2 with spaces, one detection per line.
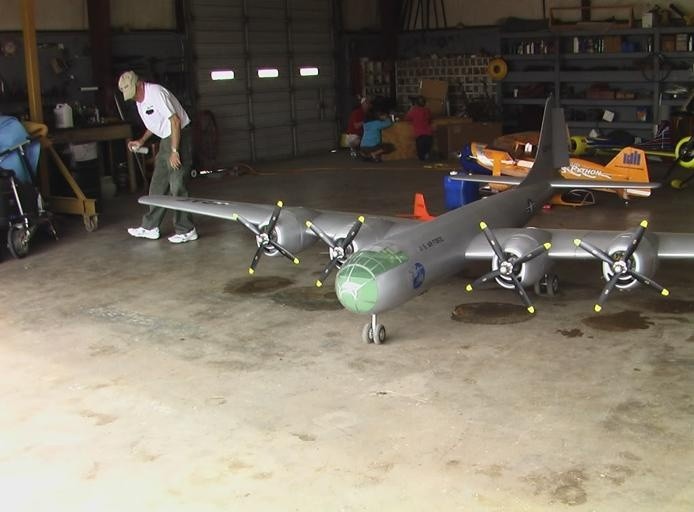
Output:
116 70 198 243
347 95 396 163
405 96 440 161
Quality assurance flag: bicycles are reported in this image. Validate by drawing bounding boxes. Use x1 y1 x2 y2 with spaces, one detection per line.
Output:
0 134 61 259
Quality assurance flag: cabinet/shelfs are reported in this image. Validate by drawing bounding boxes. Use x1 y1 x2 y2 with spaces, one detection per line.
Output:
488 28 694 190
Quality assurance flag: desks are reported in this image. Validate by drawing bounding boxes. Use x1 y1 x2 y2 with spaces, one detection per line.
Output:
369 111 480 164
40 124 137 194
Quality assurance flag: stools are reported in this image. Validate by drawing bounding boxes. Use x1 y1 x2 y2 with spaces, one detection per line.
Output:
341 127 364 163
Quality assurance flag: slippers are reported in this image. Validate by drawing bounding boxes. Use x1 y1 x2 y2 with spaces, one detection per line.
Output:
357 152 383 163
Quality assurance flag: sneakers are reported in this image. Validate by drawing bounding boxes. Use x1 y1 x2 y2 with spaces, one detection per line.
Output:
168 227 198 243
127 226 160 240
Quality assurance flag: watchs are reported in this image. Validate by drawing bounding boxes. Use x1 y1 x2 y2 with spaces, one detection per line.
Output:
171 146 177 152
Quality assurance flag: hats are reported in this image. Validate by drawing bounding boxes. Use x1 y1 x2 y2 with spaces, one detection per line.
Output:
408 95 425 106
118 70 138 101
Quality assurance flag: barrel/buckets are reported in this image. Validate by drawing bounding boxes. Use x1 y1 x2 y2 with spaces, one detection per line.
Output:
461 147 493 173
52 103 74 130
442 171 478 210
